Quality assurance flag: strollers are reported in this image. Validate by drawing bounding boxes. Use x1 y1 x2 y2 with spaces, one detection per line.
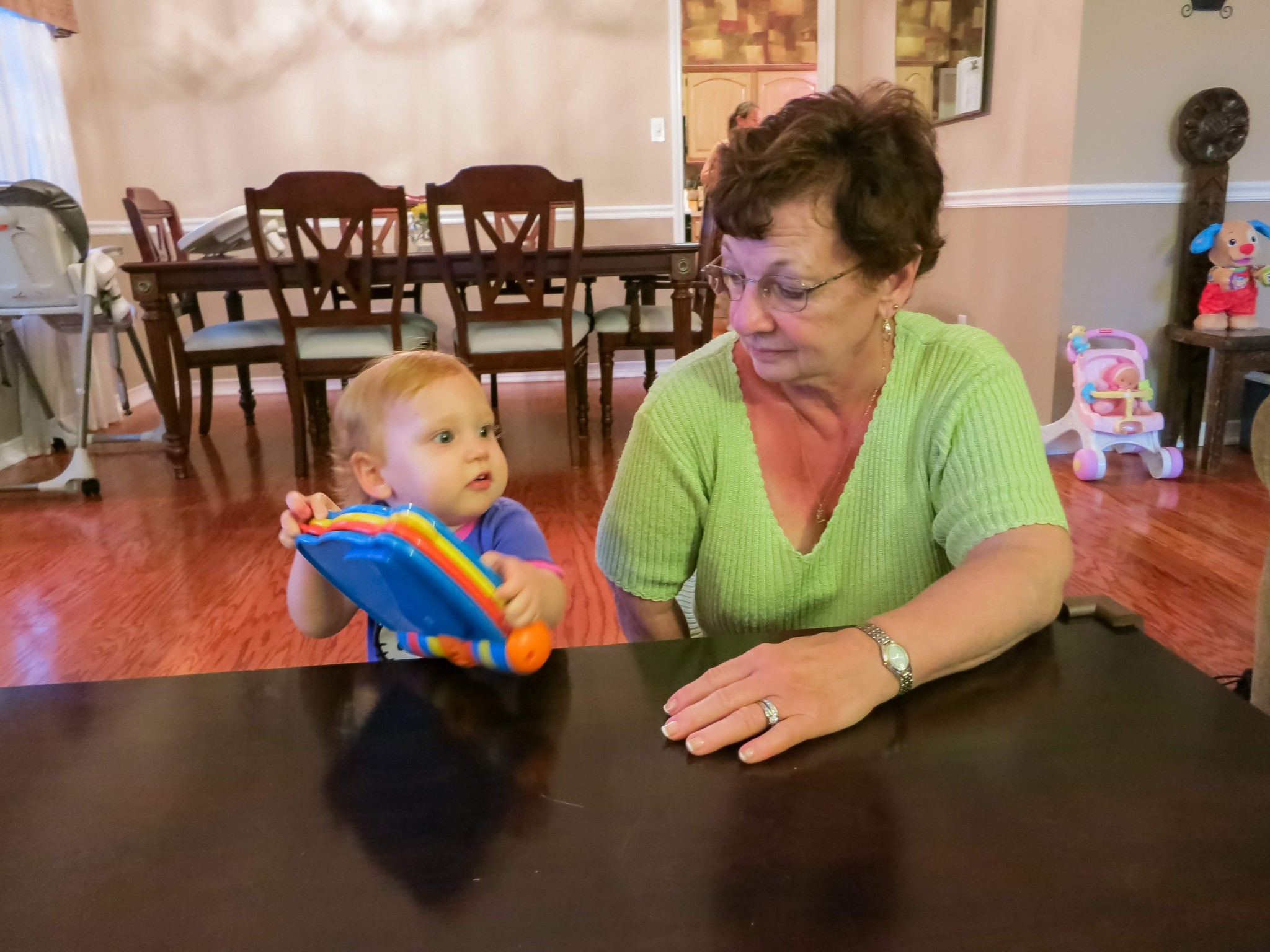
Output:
1039 323 1184 482
0 177 167 501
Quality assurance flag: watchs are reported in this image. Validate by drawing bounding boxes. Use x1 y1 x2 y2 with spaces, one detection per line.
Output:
855 623 914 695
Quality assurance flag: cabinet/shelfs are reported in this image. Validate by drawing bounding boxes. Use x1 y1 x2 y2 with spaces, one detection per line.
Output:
687 70 819 163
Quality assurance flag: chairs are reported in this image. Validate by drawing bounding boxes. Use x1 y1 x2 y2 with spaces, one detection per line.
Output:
334 182 438 391
121 184 314 447
426 164 589 467
1160 88 1270 474
245 170 430 478
596 196 722 432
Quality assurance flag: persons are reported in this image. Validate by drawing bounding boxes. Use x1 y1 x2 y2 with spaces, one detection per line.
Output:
594 77 1077 765
278 351 568 662
1093 356 1144 416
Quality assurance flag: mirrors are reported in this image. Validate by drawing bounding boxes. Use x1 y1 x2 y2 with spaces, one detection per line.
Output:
896 0 987 125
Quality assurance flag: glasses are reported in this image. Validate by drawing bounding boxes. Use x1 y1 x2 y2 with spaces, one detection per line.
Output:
701 254 865 313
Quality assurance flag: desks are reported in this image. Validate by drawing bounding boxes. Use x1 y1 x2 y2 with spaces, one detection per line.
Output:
1 584 1269 952
120 218 701 478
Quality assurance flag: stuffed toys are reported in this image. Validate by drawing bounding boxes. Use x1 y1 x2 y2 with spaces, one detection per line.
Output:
1189 220 1270 330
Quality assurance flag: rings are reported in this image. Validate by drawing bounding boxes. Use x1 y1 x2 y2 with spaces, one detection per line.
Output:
755 698 779 730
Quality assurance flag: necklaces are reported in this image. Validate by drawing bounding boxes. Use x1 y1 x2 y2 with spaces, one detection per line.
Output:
779 320 892 524
